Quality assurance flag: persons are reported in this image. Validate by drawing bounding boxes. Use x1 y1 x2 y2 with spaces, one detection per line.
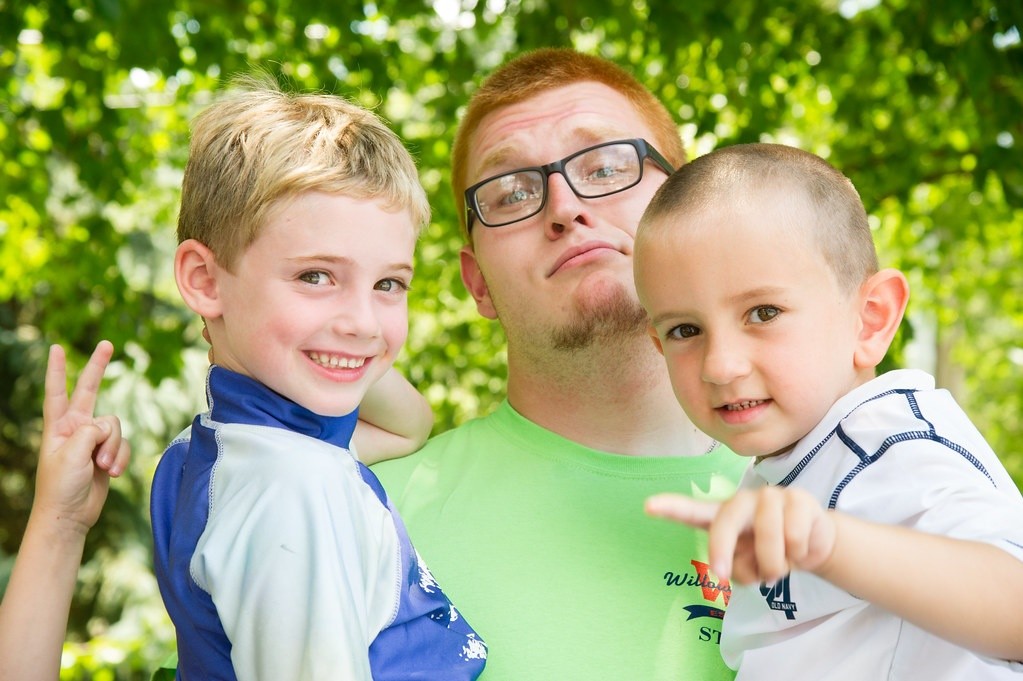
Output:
0 339 129 681
364 46 762 681
150 65 488 681
632 144 1023 679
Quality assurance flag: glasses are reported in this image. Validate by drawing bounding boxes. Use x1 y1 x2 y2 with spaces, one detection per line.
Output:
464 139 677 245
